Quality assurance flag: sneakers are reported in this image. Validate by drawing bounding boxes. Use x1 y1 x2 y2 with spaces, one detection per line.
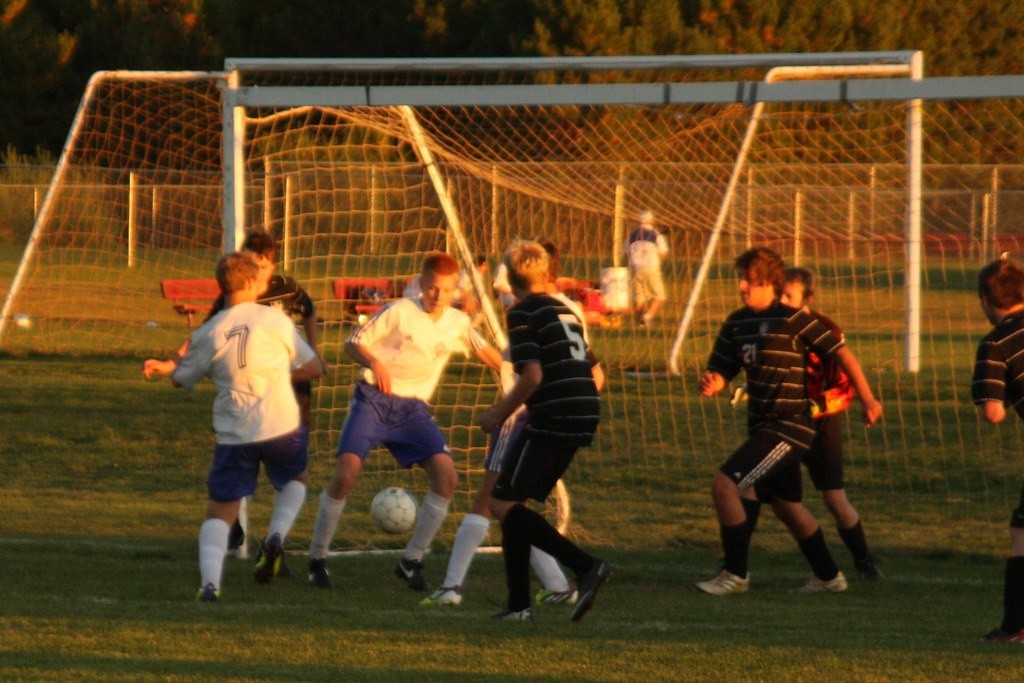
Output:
569 558 613 622
489 605 531 624
307 558 331 588
535 589 579 608
419 584 461 606
393 559 426 590
795 570 849 595
253 535 286 584
197 585 220 603
696 569 749 597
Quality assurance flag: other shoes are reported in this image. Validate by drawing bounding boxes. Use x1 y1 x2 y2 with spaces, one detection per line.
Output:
859 559 885 582
981 623 1024 647
227 521 244 547
257 548 295 581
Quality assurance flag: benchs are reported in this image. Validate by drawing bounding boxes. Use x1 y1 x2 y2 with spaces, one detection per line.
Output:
159 278 222 325
333 273 394 315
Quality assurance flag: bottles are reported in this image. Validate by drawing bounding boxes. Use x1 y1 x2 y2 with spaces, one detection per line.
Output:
2 314 33 358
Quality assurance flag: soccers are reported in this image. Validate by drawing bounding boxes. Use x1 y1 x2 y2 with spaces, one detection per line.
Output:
371 486 419 534
16 312 32 329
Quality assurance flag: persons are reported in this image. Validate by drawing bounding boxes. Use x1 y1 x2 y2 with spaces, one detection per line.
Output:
452 254 488 314
417 238 613 625
492 262 511 297
141 223 328 553
971 258 1024 646
169 250 324 602
621 210 670 329
696 247 883 599
729 268 880 581
306 250 504 590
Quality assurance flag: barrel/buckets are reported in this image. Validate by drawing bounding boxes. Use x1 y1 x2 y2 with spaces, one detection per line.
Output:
599 266 631 309
